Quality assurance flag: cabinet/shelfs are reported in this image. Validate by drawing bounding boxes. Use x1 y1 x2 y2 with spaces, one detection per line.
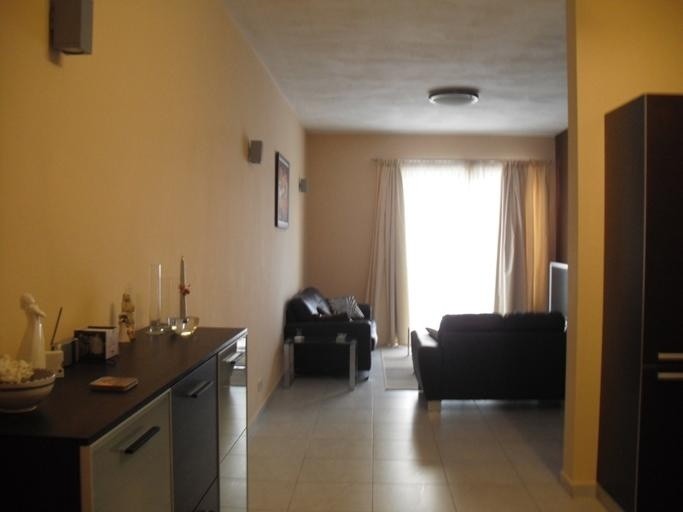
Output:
599 94 680 510
0 323 248 511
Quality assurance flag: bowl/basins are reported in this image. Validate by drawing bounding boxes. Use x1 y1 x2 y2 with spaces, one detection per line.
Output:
0 367 57 414
167 316 200 337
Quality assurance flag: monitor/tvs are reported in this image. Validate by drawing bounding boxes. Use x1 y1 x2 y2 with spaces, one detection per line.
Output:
549 262 568 329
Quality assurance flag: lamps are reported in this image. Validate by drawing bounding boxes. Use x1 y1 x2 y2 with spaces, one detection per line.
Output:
431 94 479 107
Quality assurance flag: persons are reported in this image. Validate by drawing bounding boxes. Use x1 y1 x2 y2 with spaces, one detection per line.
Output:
118 291 138 343
15 292 48 368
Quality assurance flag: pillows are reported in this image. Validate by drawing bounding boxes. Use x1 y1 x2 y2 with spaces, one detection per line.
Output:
328 296 365 319
426 326 438 341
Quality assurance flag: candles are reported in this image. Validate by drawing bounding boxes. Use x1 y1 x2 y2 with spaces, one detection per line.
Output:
180 258 189 319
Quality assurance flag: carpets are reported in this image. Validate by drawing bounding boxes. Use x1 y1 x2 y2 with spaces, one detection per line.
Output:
382 347 419 388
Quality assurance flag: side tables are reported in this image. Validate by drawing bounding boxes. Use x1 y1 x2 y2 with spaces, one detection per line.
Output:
282 337 356 389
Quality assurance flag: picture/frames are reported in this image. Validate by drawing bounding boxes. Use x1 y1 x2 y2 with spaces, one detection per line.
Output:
274 152 289 227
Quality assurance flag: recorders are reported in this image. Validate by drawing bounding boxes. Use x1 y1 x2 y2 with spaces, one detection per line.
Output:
51 307 80 367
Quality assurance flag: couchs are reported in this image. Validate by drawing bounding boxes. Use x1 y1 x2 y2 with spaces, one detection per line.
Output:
285 287 378 375
411 313 565 412
